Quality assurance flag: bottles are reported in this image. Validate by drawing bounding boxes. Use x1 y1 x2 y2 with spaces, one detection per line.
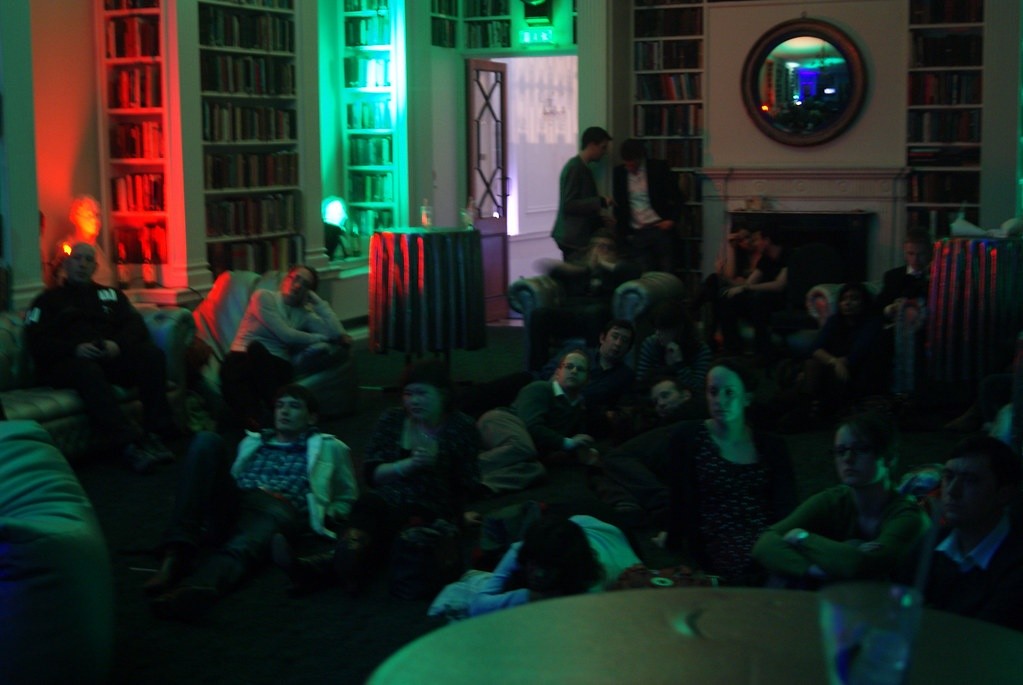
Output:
139 239 158 290
114 243 133 289
421 199 433 229
458 197 477 229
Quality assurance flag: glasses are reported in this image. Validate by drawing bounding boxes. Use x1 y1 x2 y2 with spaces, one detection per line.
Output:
829 445 881 460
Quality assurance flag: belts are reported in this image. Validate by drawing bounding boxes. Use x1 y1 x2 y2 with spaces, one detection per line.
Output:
259 487 294 510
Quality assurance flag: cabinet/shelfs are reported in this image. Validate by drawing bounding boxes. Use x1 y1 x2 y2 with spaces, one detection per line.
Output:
29 1 304 307
631 0 706 280
334 0 396 253
906 0 985 258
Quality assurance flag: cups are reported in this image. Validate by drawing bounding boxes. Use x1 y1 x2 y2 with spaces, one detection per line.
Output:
815 592 919 685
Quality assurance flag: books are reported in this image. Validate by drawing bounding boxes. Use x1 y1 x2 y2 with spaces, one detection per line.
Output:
634 0 703 232
104 0 390 282
909 0 983 250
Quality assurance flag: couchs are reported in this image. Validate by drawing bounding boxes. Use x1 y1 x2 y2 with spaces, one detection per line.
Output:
508 257 682 377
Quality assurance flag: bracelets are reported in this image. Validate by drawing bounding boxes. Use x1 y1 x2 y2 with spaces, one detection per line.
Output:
742 285 747 290
394 459 408 477
828 358 836 362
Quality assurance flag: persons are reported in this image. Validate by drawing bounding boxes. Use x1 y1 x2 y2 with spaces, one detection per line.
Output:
547 126 616 261
611 137 685 273
0 224 1023 685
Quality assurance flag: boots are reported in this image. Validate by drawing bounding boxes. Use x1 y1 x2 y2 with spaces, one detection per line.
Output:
333 527 370 600
285 547 340 598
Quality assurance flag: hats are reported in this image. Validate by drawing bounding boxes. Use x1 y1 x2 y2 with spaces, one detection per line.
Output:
400 358 448 389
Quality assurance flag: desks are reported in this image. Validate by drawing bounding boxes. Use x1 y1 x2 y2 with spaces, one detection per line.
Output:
369 225 484 356
361 586 1022 684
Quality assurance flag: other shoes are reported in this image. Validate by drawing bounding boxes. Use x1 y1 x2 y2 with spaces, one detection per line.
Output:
153 585 209 619
145 434 175 465
120 445 159 477
142 575 171 593
238 415 260 437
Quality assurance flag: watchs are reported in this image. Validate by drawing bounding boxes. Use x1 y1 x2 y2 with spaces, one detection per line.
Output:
792 530 812 546
